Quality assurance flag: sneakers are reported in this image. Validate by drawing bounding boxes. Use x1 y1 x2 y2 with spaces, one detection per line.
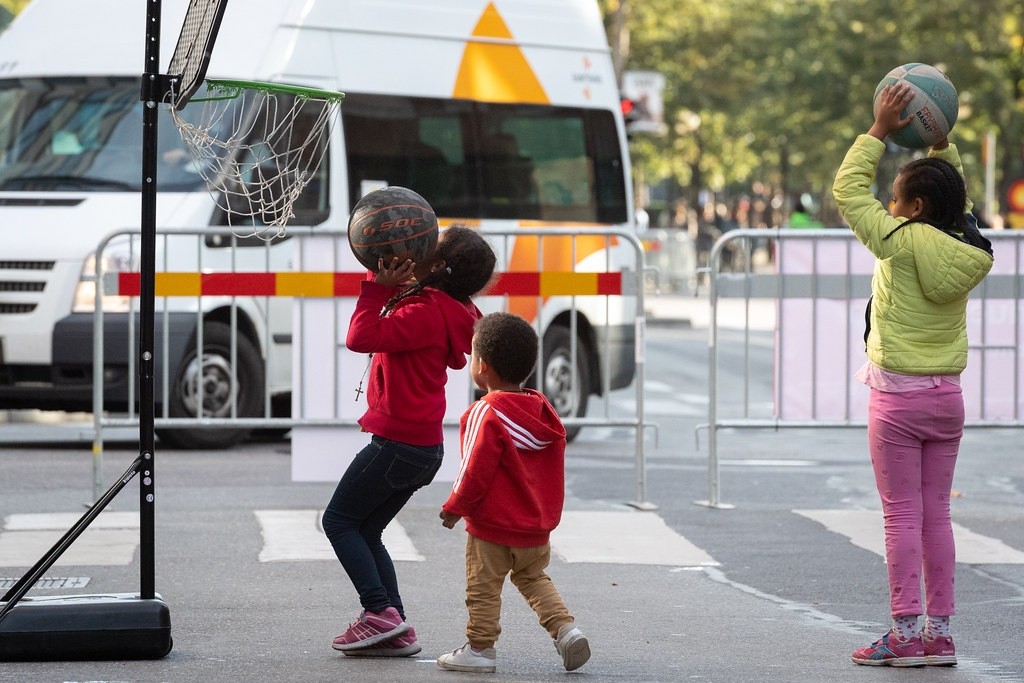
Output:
553 622 591 672
436 642 496 674
331 605 411 651
915 635 959 667
851 630 926 667
340 623 423 657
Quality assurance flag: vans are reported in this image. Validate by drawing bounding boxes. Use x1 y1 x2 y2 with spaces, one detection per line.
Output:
0 0 640 447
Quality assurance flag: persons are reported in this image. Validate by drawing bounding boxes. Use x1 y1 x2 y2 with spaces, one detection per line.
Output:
163 100 274 190
787 202 825 229
437 312 592 673
321 224 498 658
832 81 995 668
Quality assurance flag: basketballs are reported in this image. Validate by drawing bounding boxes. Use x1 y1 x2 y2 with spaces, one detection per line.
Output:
347 185 439 275
873 62 958 149
